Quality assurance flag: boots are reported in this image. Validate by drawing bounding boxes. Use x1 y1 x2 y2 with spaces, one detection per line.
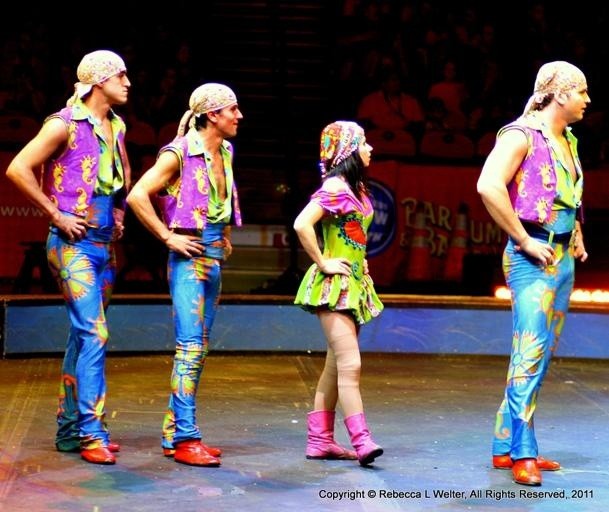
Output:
303 409 358 460
342 412 385 467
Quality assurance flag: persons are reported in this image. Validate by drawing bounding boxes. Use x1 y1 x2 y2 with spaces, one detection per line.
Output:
340 0 609 166
476 60 593 487
4 49 131 464
124 82 245 468
292 120 385 466
0 22 191 147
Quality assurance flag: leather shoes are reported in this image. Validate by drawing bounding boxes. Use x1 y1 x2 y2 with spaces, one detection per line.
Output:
511 458 542 488
81 441 119 465
162 439 221 467
492 451 562 471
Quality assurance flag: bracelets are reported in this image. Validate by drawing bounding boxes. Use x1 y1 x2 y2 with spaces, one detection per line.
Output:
162 229 174 241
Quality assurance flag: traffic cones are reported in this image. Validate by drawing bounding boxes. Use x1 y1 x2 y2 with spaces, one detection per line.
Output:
400 198 437 286
443 200 479 288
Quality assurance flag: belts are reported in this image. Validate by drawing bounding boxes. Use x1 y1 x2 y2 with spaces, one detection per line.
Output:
524 222 573 244
